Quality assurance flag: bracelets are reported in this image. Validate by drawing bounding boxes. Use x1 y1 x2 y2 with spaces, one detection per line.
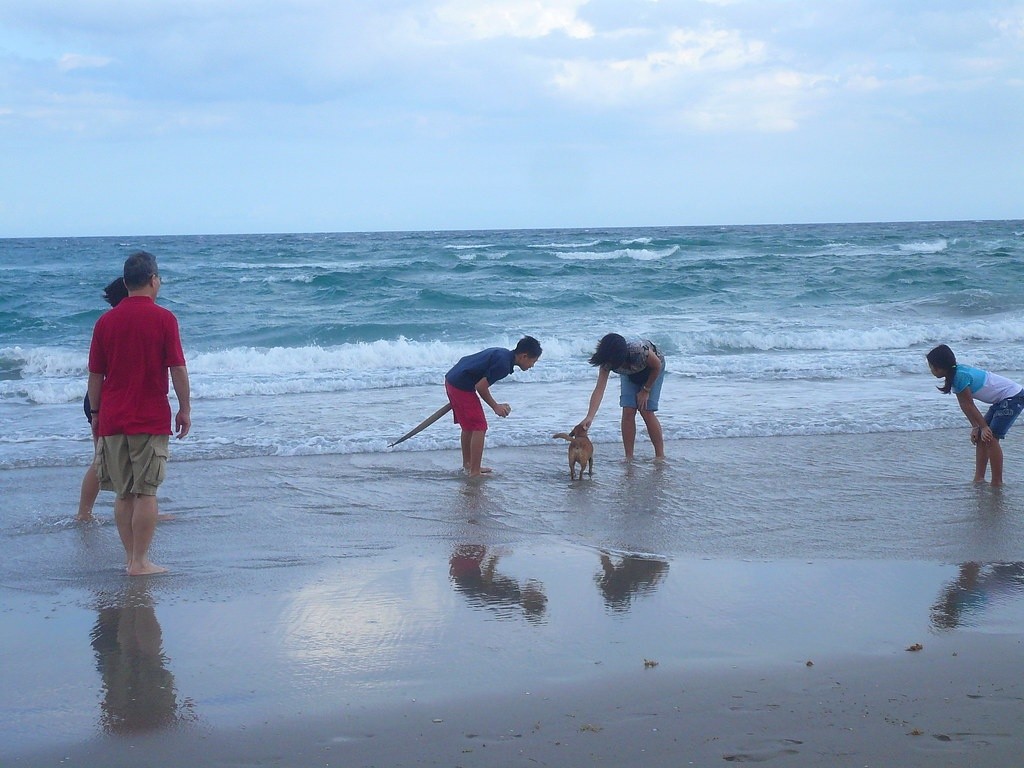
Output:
972 426 979 430
643 386 651 392
89 409 99 414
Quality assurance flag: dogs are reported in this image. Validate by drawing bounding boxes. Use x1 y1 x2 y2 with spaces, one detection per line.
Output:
552 422 594 479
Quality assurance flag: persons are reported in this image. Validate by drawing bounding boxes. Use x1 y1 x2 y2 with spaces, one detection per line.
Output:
93 578 174 732
931 561 1024 631
574 334 668 463
450 486 548 617
86 252 192 576
74 276 175 525
926 344 1024 487
596 547 671 606
445 337 543 479
